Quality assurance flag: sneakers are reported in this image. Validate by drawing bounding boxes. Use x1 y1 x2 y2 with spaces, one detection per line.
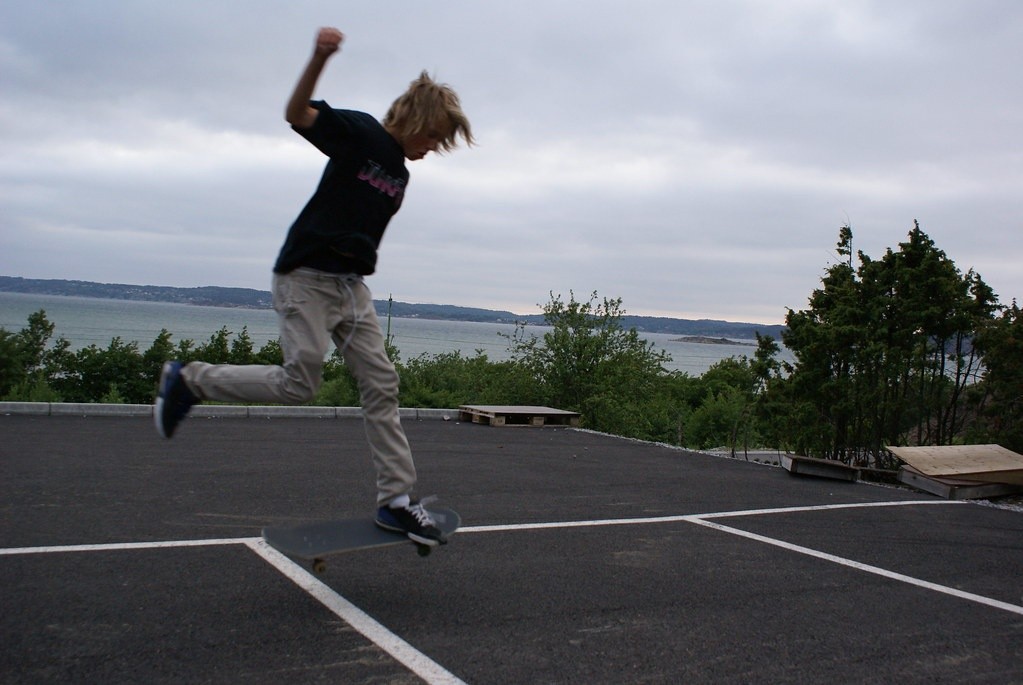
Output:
154 362 200 440
374 491 448 546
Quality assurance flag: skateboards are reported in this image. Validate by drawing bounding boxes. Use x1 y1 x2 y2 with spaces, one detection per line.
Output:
259 504 463 576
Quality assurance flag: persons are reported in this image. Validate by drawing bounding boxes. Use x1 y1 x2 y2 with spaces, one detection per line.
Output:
154 28 481 547
